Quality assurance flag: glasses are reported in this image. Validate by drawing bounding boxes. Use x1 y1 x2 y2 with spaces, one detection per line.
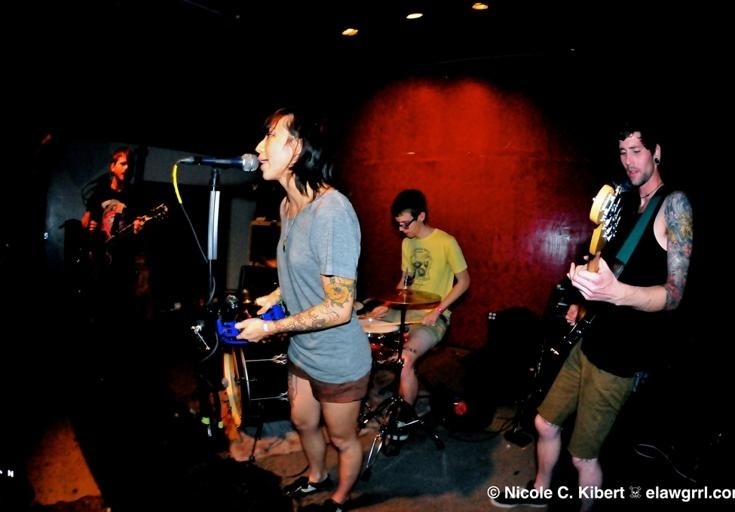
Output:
396 218 415 227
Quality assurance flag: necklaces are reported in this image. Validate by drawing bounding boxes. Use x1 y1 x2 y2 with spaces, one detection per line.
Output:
280 204 303 255
638 183 664 198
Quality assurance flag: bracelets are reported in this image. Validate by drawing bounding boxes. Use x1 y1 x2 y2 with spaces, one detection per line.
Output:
436 305 445 314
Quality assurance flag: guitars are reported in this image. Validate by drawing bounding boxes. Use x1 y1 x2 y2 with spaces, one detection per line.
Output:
89 202 170 256
541 182 625 365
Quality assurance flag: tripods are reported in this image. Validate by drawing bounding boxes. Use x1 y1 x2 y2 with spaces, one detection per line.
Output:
354 308 446 479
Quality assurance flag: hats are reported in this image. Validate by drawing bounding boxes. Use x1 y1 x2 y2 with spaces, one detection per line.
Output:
489 479 548 509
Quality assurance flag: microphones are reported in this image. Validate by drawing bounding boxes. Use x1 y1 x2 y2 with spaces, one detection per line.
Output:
179 153 259 172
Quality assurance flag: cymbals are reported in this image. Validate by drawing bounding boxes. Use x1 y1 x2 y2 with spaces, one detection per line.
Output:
368 287 441 308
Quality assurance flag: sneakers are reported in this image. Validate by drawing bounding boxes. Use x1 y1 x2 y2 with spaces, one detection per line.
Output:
281 472 350 512
382 403 419 442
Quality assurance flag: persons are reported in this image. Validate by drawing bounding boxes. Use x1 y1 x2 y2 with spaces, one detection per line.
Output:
483 112 698 512
228 102 374 510
363 185 472 408
73 147 154 297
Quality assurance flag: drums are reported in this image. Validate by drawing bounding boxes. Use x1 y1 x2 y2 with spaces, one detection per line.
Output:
222 337 289 427
354 298 366 316
358 317 401 363
